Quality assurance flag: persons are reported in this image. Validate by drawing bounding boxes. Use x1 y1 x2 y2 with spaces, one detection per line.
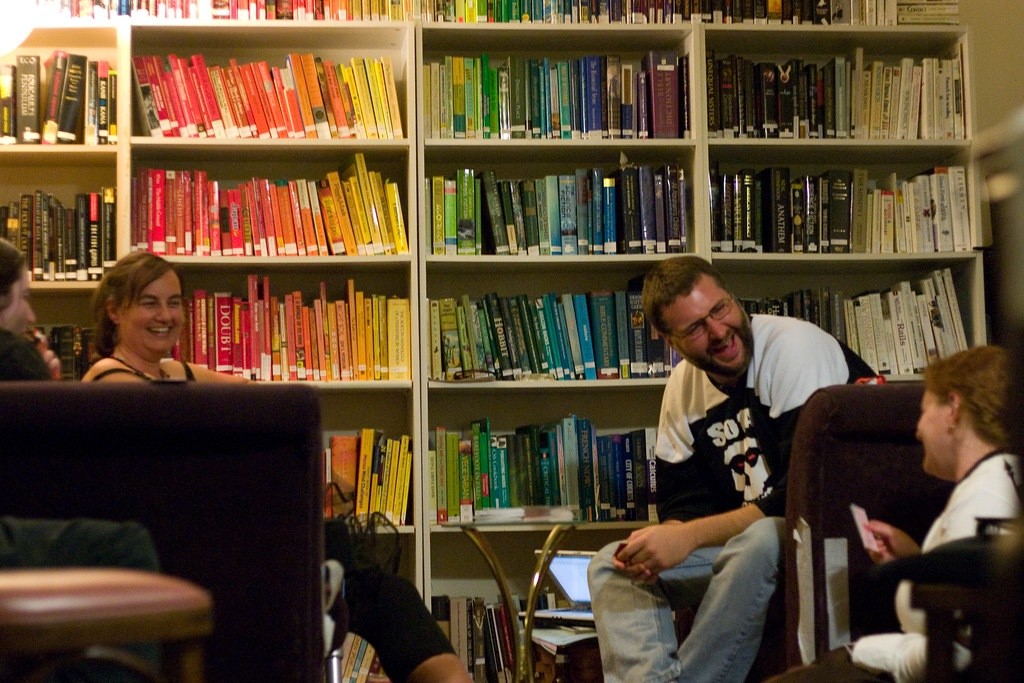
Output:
81 250 476 683
0 236 62 381
587 256 879 683
767 345 1024 683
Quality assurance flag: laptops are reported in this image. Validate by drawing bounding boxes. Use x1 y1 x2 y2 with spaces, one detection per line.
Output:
518 549 598 628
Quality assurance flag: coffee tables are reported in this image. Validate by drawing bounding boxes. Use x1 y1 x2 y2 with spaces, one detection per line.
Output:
442 506 584 683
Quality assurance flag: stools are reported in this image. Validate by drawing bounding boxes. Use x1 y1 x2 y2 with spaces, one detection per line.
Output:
0 565 213 683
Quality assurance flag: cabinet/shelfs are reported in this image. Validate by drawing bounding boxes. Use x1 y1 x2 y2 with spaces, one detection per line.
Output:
0 21 987 619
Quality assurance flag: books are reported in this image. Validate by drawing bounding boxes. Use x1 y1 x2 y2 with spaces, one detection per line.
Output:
323 415 656 683
0 0 966 278
36 270 968 383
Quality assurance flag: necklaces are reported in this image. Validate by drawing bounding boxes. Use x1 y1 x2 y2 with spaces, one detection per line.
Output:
109 352 171 382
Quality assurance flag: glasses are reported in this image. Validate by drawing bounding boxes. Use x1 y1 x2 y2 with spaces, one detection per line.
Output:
668 288 734 343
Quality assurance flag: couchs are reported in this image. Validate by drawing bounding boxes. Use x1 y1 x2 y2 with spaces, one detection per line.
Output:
675 379 957 683
0 382 352 683
